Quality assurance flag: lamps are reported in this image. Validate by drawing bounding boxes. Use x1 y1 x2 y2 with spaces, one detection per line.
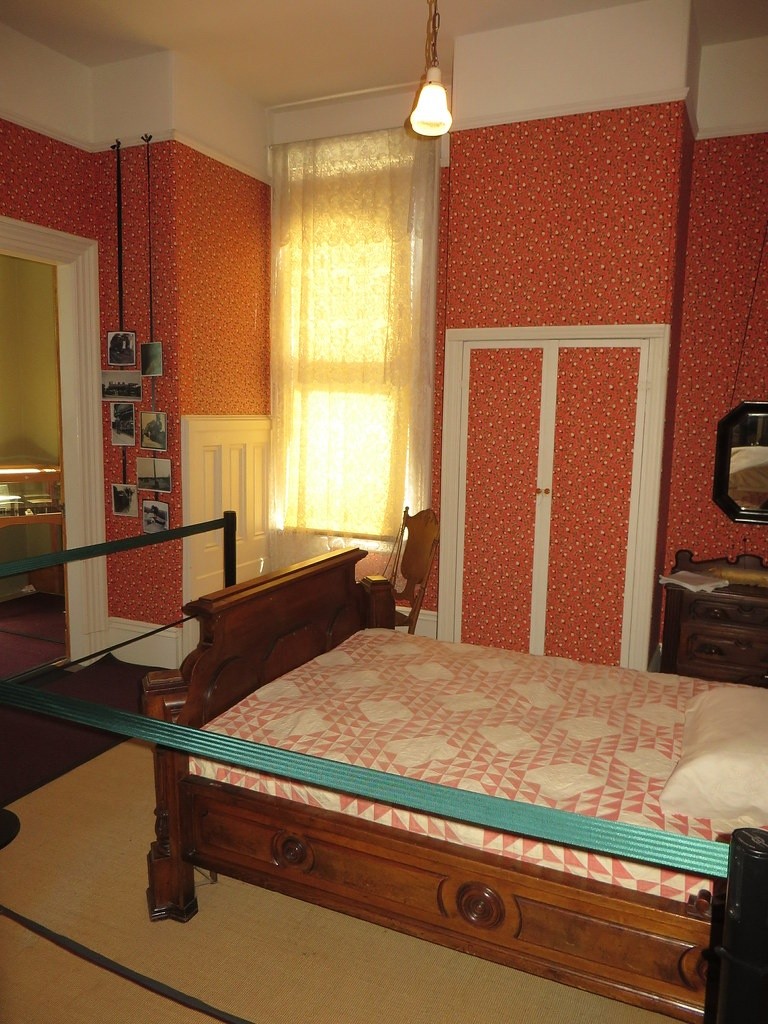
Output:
410 0 453 137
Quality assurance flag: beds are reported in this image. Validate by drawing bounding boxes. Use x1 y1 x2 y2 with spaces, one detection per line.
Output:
141 546 768 1024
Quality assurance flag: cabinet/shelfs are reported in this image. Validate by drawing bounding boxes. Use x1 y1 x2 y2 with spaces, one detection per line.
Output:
660 549 768 688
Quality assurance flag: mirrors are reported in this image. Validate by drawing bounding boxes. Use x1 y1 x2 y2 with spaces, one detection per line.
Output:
711 401 768 525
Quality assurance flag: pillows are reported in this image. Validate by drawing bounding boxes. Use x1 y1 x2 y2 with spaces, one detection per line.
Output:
659 686 768 833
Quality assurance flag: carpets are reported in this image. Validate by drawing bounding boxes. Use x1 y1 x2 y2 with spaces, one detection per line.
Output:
0 592 164 808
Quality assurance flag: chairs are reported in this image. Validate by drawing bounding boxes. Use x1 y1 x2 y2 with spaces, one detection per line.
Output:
382 506 440 634
